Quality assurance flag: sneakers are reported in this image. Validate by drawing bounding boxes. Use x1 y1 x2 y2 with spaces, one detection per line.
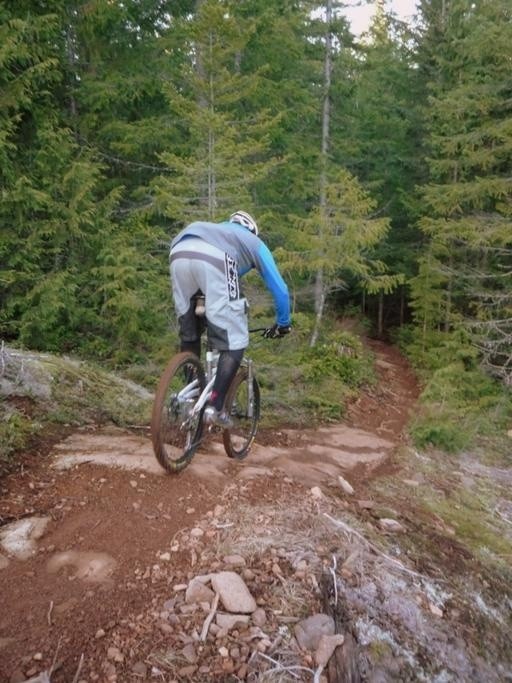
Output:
201 406 236 428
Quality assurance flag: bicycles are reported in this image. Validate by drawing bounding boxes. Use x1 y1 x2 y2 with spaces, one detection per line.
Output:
150 295 291 475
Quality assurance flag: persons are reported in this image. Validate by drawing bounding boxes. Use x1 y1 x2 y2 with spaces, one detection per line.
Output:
168 209 294 431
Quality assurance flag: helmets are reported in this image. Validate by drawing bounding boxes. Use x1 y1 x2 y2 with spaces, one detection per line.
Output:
229 208 260 235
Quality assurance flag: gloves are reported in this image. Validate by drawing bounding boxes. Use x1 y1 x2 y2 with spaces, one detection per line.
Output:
260 322 285 339
